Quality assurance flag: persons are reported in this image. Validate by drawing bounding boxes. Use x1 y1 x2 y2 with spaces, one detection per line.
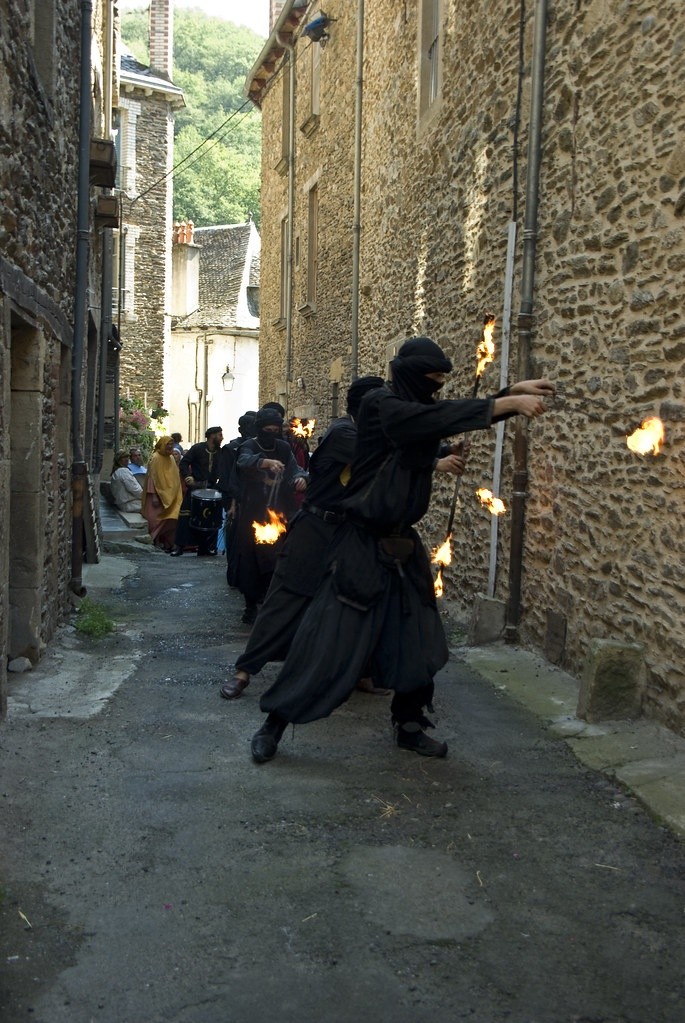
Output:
172 432 191 474
128 448 146 489
250 338 556 761
141 436 195 552
170 426 223 557
219 376 471 698
219 402 309 625
110 449 143 512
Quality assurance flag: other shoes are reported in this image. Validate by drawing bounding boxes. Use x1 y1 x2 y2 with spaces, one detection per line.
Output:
242 608 257 625
396 728 448 759
171 547 183 556
220 670 250 699
251 714 287 763
198 548 215 556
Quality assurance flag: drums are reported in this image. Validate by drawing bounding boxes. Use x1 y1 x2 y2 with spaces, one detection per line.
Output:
188 487 223 531
